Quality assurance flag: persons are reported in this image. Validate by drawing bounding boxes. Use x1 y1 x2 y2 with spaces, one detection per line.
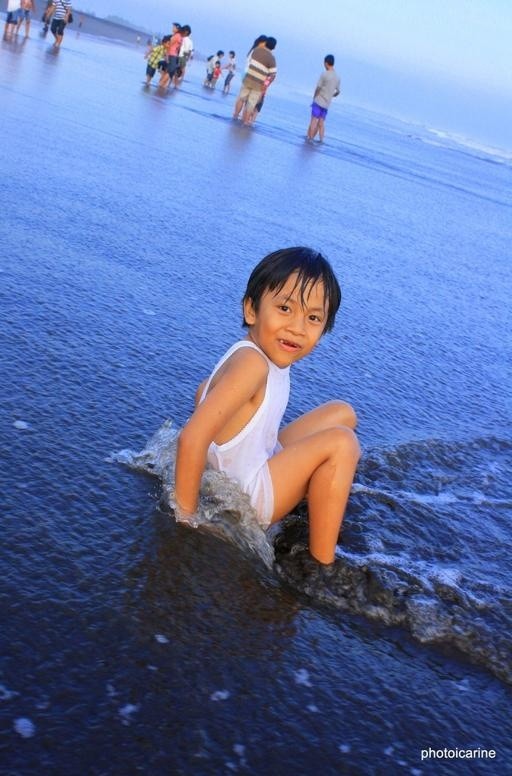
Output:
2 0 84 48
174 246 362 567
141 22 196 94
202 35 278 129
307 54 340 143
